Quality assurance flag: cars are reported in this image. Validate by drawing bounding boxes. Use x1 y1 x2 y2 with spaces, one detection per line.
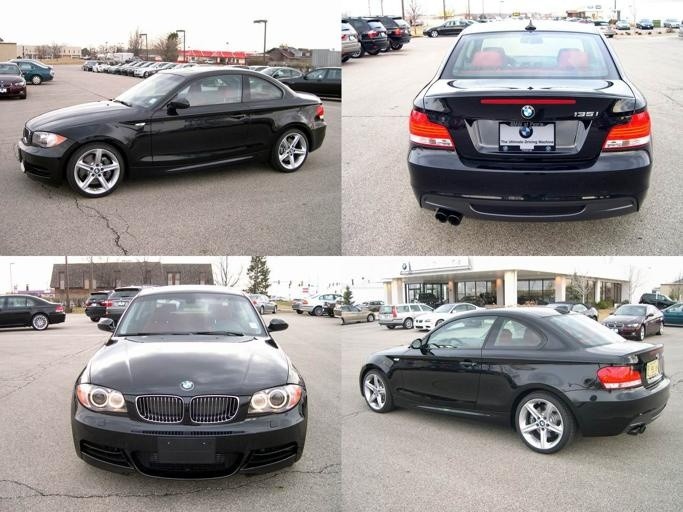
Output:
68 285 310 481
342 299 486 331
359 307 671 455
16 65 325 198
422 18 630 38
635 18 655 30
0 294 65 331
547 301 598 322
412 293 554 306
663 19 680 29
291 293 341 319
409 18 652 226
658 302 682 327
0 56 53 99
598 303 665 341
240 293 277 315
80 58 340 102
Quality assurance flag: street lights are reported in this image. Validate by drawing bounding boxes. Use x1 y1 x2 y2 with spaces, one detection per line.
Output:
138 34 148 61
175 30 185 62
253 20 266 62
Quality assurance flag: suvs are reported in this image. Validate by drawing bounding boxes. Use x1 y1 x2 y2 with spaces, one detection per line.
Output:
340 16 412 64
638 292 677 308
85 285 180 326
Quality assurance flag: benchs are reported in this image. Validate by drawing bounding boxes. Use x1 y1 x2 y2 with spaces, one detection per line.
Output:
467 49 594 77
